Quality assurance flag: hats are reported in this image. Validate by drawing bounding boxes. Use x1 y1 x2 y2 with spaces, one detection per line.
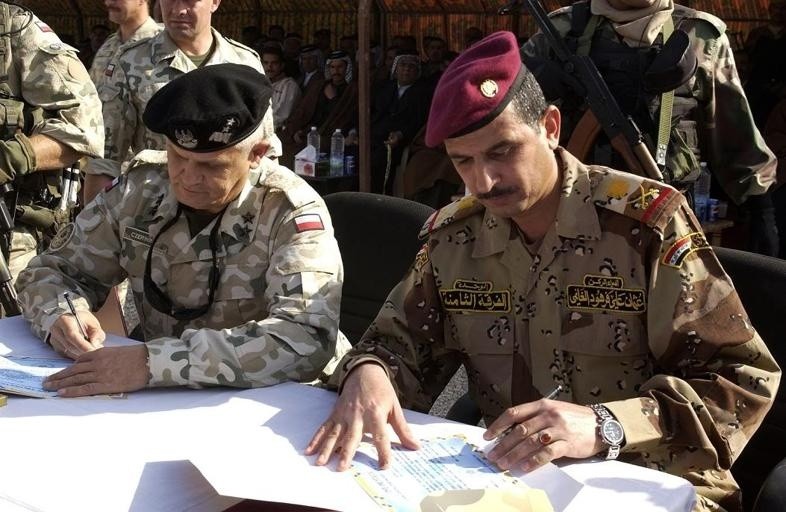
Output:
142 63 274 151
426 31 526 147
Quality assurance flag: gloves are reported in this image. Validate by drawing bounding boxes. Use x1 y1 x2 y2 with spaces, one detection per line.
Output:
749 193 779 257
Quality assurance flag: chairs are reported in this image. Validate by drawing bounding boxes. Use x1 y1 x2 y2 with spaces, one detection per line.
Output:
322 189 435 342
708 244 786 512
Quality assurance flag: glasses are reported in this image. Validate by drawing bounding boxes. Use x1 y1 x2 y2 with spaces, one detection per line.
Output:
145 205 225 321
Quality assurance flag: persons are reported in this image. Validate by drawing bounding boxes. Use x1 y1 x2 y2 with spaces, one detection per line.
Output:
1 1 105 317
84 0 282 208
239 25 489 199
306 33 782 510
518 1 779 256
14 64 353 397
89 0 166 85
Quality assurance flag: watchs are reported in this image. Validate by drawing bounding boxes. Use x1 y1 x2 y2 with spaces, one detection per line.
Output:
589 404 623 462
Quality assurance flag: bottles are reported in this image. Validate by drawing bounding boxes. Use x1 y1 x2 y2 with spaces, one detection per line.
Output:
709 199 719 222
329 129 345 178
306 126 320 163
693 161 710 223
345 133 358 178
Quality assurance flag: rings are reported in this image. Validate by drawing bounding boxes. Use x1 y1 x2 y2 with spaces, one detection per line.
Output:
539 430 551 448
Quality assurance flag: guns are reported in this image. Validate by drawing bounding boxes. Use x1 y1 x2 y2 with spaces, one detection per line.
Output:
0 183 19 315
525 2 668 183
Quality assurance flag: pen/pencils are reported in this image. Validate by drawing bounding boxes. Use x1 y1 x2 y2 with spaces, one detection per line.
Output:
493 385 564 445
61 290 92 343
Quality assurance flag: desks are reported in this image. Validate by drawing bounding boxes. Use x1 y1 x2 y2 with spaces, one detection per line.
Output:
1 312 697 512
276 149 359 196
